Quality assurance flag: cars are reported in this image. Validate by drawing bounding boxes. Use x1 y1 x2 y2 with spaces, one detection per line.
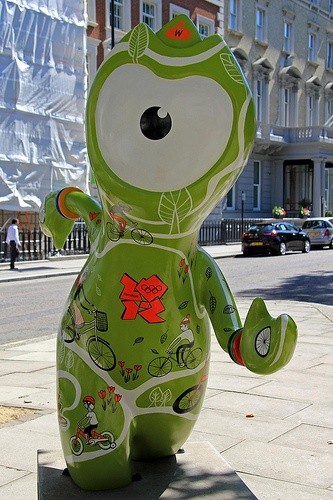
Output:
301 217 333 251
240 221 311 256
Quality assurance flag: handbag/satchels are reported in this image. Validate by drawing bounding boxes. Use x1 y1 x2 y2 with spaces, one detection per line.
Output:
7 245 12 258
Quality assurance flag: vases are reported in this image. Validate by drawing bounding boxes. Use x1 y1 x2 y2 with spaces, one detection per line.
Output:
299 216 308 219
275 215 285 219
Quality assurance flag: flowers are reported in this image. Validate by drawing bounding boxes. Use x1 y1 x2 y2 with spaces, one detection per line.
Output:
272 206 286 215
299 206 311 216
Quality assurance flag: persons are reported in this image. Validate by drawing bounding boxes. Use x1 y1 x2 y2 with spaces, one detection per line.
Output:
6 218 22 270
